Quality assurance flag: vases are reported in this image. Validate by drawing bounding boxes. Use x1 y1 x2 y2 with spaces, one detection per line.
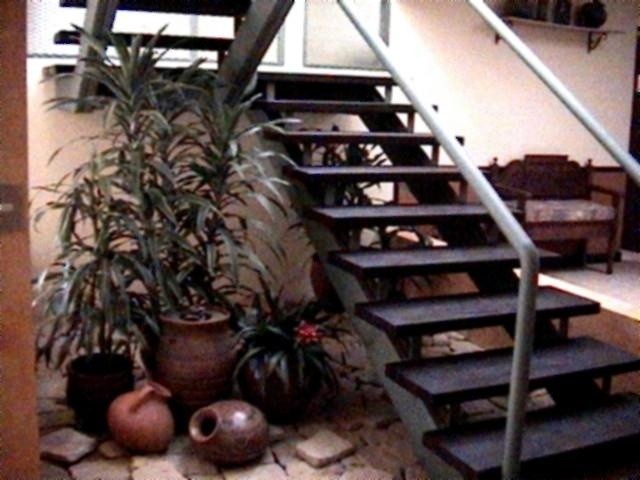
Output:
236 362 323 421
66 349 271 469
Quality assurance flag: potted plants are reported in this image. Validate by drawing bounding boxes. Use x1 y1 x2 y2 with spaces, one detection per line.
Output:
30 16 302 424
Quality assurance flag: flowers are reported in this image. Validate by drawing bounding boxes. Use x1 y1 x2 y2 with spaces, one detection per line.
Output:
232 290 352 391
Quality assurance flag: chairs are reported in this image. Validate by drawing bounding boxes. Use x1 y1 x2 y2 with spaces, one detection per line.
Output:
491 152 626 277
